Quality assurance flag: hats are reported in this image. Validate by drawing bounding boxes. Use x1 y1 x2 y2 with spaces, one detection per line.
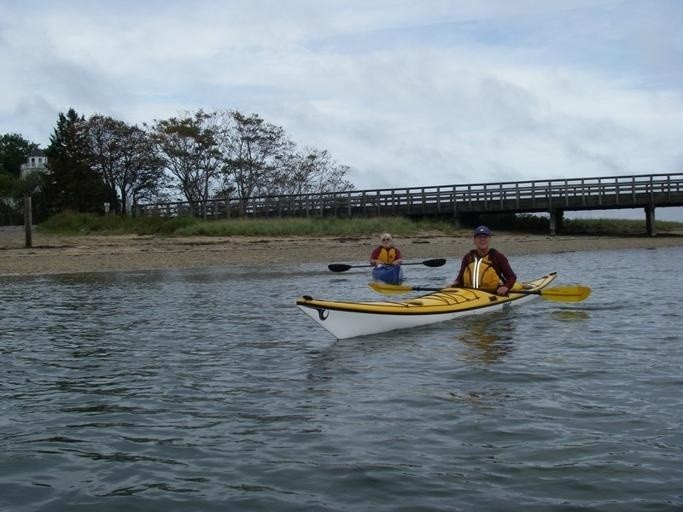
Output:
474 226 490 236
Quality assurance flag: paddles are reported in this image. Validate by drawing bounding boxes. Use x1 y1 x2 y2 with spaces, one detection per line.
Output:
327 259 446 272
367 281 592 302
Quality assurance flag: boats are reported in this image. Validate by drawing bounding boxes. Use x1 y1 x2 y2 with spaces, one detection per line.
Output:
370 263 404 283
293 271 566 342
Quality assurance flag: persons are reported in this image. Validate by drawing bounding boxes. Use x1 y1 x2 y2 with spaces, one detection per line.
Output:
369 233 404 269
442 226 516 297
